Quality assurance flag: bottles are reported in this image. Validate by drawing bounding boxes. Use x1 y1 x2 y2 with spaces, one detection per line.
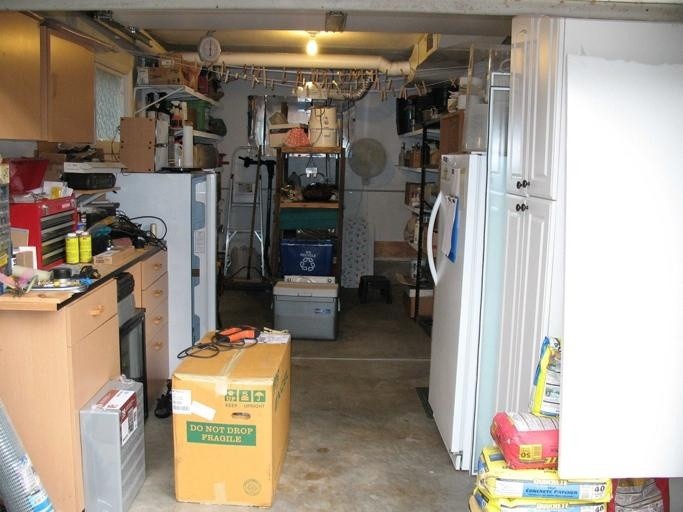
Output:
399 142 439 167
65 230 91 264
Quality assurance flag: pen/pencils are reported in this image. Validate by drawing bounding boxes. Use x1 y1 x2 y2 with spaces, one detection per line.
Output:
27 275 39 293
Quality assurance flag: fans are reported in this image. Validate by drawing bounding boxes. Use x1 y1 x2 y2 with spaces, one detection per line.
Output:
347 138 386 178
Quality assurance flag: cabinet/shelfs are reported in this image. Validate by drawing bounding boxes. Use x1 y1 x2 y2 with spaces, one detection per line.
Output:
269 146 344 289
133 82 222 170
492 12 682 448
0 10 94 144
392 126 440 319
106 169 218 380
0 242 169 512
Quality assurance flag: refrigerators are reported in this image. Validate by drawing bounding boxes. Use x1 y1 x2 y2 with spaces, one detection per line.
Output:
425 150 484 470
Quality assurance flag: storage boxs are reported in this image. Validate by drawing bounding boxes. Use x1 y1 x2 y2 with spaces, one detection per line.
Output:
77 376 146 512
271 280 339 339
169 332 290 505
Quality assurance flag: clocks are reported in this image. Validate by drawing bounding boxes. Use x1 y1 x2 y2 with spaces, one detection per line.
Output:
197 36 221 63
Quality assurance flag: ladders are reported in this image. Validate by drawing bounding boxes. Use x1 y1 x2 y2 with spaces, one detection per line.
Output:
224 146 264 277
